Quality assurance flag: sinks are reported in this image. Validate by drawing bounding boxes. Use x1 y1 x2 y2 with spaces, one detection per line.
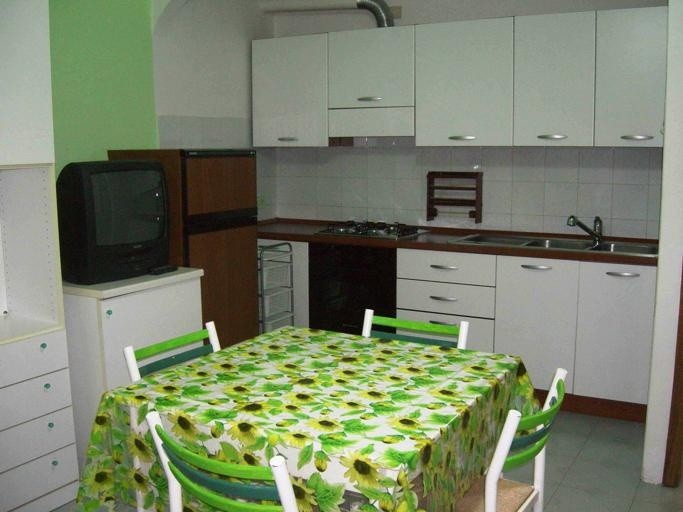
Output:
591 242 658 257
524 238 595 251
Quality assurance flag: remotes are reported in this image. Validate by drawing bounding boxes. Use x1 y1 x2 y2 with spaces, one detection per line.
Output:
150 264 175 276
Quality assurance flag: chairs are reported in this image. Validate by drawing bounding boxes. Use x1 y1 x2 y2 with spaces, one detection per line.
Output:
123 319 221 381
361 309 469 350
146 409 299 510
453 367 569 511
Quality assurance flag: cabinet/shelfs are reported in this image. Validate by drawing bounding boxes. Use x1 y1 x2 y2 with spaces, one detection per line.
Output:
0 0 79 510
396 247 496 354
61 266 205 484
496 255 657 407
252 33 354 148
414 16 513 146
513 6 668 148
257 242 295 333
327 25 414 139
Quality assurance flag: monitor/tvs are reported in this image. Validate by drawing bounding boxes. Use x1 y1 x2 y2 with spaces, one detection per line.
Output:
56 159 168 285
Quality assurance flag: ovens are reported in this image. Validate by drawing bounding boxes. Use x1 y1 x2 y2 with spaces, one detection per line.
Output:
308 243 396 337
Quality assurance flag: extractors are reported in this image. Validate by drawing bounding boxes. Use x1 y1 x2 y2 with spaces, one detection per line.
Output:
328 136 414 148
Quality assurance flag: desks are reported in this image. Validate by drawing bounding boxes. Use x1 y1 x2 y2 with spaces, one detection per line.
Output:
76 325 541 511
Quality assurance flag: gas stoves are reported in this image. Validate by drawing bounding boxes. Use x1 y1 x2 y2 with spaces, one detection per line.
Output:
314 220 428 241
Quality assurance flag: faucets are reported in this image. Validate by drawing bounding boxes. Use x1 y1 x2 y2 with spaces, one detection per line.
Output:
567 213 602 241
